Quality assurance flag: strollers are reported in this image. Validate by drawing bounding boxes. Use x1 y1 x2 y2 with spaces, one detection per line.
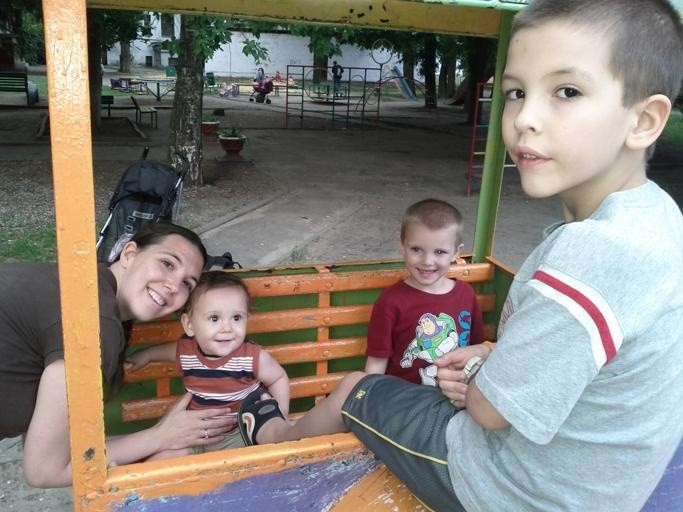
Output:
248 68 276 105
93 139 194 263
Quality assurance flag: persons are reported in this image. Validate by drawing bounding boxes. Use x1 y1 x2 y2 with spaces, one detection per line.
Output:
332 61 344 96
275 71 281 82
1 225 237 489
126 271 291 462
288 75 294 84
366 199 484 386
238 1 683 512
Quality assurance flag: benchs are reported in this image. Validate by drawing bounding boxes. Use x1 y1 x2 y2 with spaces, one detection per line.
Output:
128 94 159 130
231 82 302 96
100 95 115 116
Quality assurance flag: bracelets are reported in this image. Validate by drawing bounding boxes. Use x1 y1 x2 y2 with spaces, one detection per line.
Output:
485 340 495 357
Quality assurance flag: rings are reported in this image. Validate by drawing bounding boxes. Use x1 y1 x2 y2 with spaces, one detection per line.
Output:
205 430 209 439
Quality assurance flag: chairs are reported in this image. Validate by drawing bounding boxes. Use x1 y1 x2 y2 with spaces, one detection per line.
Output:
0 68 35 106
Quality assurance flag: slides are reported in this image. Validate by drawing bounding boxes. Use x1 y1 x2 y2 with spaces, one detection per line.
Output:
383 64 417 101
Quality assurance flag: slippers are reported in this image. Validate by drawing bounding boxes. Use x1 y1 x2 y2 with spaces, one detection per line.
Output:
237 390 286 447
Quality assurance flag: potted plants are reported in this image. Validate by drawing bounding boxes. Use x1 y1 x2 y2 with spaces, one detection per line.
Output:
216 122 249 157
199 110 219 135
